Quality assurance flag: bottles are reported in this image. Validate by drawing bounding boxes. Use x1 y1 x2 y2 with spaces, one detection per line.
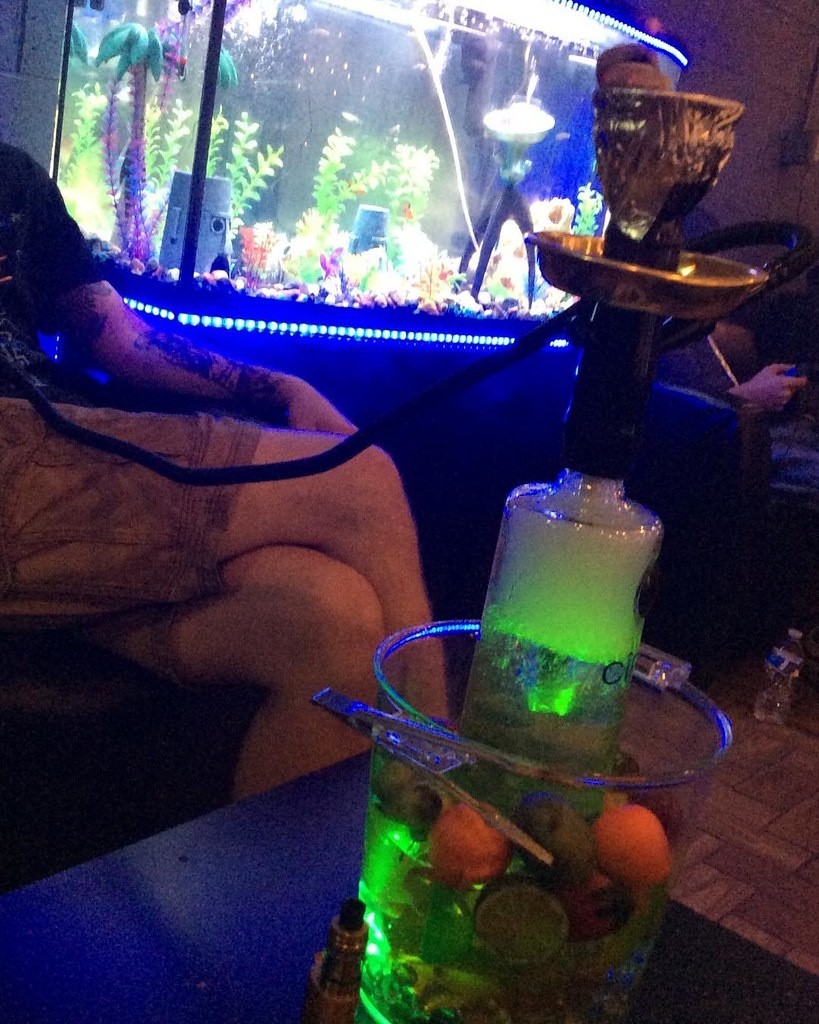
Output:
461 220 685 877
753 628 804 725
320 898 368 996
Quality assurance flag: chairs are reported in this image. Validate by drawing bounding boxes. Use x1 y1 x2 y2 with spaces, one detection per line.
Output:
0 625 266 897
621 384 819 693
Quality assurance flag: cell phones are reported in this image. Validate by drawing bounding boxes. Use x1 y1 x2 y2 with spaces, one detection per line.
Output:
784 362 810 379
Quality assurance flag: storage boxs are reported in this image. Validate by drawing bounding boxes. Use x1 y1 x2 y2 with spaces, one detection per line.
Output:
53 0 689 353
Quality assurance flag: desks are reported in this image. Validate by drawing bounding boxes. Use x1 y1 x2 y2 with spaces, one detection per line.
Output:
0 747 819 1024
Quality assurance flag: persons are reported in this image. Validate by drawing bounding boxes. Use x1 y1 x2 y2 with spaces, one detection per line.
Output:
0 141 454 803
641 206 817 691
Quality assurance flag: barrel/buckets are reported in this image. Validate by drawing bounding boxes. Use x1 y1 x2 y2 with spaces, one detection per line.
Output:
359 619 732 1023
